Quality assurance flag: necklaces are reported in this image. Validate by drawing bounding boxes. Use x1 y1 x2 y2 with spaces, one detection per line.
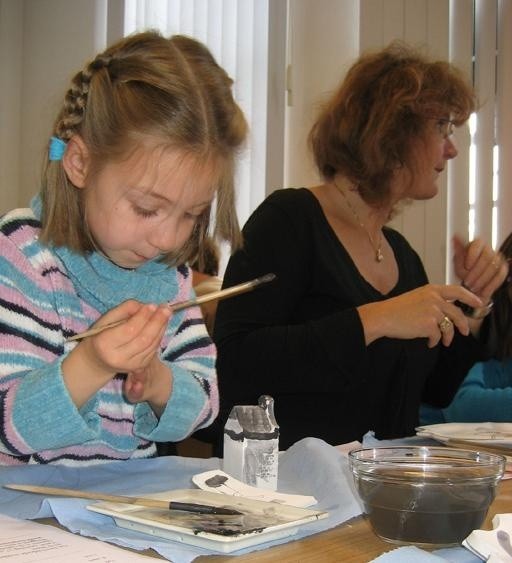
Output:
334 183 385 262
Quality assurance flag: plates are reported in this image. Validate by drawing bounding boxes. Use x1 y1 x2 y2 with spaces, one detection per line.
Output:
86 487 329 553
414 421 512 449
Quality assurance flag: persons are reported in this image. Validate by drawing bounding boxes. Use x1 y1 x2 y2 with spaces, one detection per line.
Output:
418 232 512 426
190 40 511 459
185 232 226 332
0 29 249 466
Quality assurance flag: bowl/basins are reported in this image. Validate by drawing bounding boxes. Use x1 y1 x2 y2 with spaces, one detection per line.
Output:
347 444 506 551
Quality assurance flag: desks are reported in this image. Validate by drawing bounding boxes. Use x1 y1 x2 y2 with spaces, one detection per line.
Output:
0 437 512 562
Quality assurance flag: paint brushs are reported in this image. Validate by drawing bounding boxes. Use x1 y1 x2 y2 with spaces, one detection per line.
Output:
3 482 243 516
63 274 277 340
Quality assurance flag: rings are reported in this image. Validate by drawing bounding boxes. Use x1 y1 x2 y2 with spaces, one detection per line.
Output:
439 314 451 333
491 260 500 270
